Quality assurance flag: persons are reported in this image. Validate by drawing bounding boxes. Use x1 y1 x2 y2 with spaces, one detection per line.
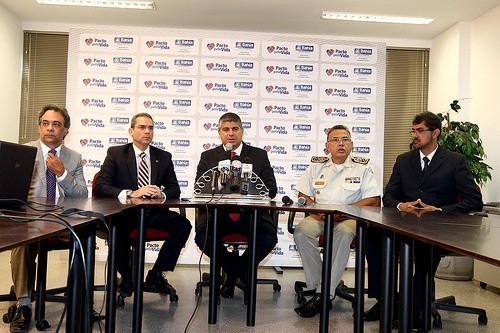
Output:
293 125 381 317
10 104 88 333
195 113 278 299
364 111 483 329
94 113 192 295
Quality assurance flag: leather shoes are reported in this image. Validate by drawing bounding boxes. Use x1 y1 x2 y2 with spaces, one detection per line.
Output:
92 308 103 320
146 270 176 294
294 294 332 318
429 306 442 328
220 271 237 298
117 282 133 297
10 306 32 332
352 300 381 321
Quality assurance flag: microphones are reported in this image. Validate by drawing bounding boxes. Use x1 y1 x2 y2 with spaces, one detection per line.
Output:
297 197 308 205
282 196 293 204
212 142 252 195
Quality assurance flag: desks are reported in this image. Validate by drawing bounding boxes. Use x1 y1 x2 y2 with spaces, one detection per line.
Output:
0 198 500 333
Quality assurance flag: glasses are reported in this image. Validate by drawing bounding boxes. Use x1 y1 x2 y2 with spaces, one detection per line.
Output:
329 138 350 145
409 130 435 136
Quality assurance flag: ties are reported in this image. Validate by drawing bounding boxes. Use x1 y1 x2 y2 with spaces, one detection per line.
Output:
46 148 57 197
138 152 150 189
423 156 430 175
228 151 240 222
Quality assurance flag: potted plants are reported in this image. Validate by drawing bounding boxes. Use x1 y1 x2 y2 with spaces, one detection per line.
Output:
403 100 492 281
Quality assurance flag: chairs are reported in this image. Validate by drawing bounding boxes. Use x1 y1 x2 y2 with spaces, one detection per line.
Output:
0 240 70 331
87 172 178 312
194 201 281 298
288 210 359 311
376 183 488 330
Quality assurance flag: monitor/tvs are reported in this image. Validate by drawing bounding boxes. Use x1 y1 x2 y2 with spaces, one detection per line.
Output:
0 140 37 208
